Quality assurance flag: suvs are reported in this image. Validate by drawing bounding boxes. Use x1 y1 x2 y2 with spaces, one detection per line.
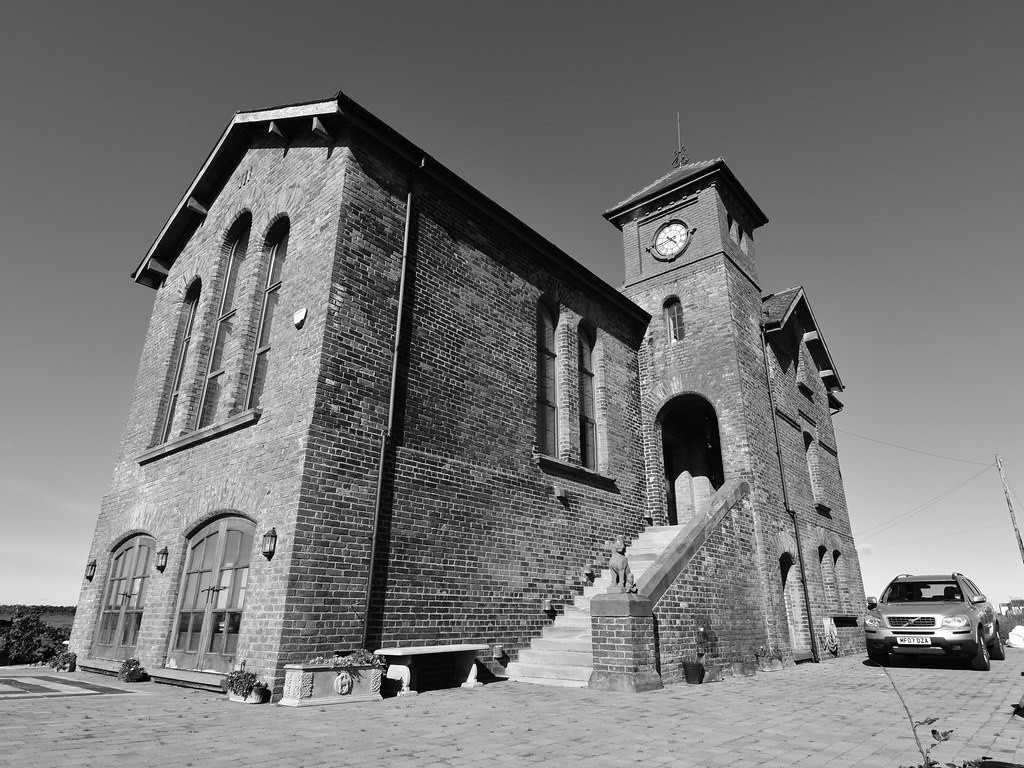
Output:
863 572 1006 671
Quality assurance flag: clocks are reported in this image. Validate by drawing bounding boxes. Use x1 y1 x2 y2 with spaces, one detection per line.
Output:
655 223 687 256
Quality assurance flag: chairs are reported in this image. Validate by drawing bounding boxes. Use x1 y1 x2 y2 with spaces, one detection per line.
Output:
933 586 956 600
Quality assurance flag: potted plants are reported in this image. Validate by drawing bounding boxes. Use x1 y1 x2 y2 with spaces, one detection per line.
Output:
277 648 387 707
48 650 77 672
680 646 784 684
220 670 268 704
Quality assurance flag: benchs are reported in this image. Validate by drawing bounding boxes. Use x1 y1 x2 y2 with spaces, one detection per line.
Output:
373 644 490 698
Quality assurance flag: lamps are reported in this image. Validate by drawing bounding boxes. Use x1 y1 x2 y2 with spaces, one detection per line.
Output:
155 546 169 573
85 559 97 582
262 527 277 560
